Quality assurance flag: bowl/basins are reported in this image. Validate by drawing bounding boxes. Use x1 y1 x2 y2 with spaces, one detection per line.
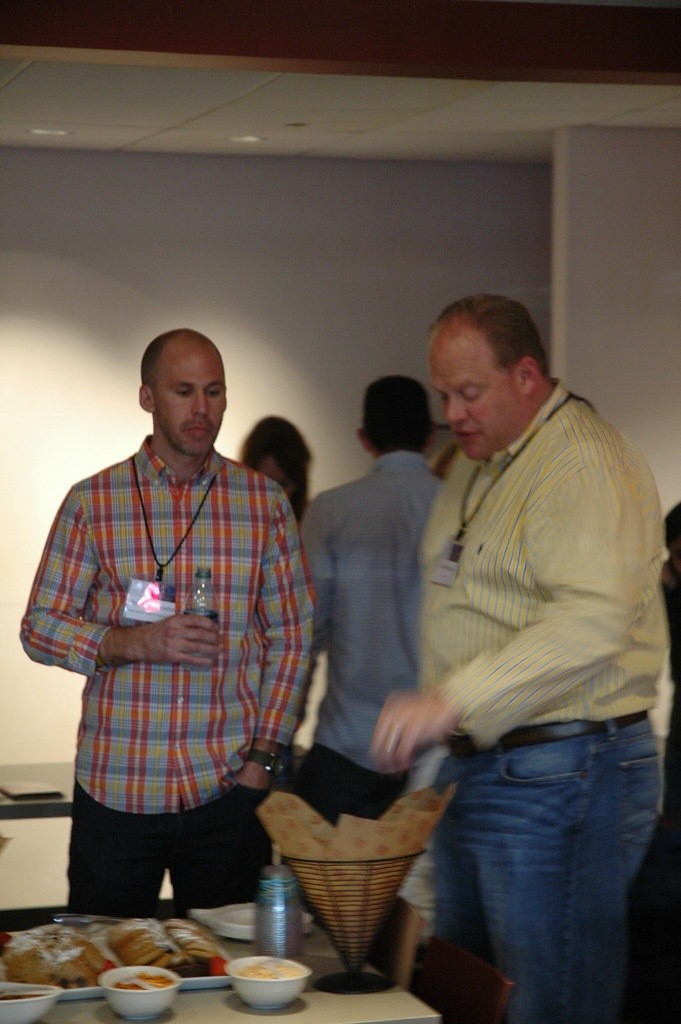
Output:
223 955 315 1008
0 982 64 1024
96 964 183 1021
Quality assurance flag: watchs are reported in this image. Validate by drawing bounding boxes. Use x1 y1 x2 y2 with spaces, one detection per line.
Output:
246 749 286 779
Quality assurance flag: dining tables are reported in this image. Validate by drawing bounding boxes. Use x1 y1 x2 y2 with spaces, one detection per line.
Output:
34 922 442 1024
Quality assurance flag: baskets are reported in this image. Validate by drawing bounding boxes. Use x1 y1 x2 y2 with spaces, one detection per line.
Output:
281 849 428 994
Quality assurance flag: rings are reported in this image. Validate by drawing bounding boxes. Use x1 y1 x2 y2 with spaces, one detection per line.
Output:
384 738 398 752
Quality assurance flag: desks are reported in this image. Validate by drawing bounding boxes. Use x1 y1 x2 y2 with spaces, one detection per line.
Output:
0 762 75 819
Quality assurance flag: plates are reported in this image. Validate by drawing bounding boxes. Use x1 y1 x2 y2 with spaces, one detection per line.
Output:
188 901 313 942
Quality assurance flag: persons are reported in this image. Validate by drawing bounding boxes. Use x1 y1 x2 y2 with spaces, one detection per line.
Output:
20 328 314 907
295 370 449 831
366 292 667 1024
662 503 681 703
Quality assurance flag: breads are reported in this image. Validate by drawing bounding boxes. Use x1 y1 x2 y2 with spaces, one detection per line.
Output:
3 918 219 988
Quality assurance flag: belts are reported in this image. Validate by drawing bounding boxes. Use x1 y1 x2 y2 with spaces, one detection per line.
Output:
447 710 648 757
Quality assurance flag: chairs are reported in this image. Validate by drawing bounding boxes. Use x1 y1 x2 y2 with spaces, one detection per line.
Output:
367 895 516 1024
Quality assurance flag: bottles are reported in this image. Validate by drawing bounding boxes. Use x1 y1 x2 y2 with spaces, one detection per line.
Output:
254 865 303 960
178 564 219 670
